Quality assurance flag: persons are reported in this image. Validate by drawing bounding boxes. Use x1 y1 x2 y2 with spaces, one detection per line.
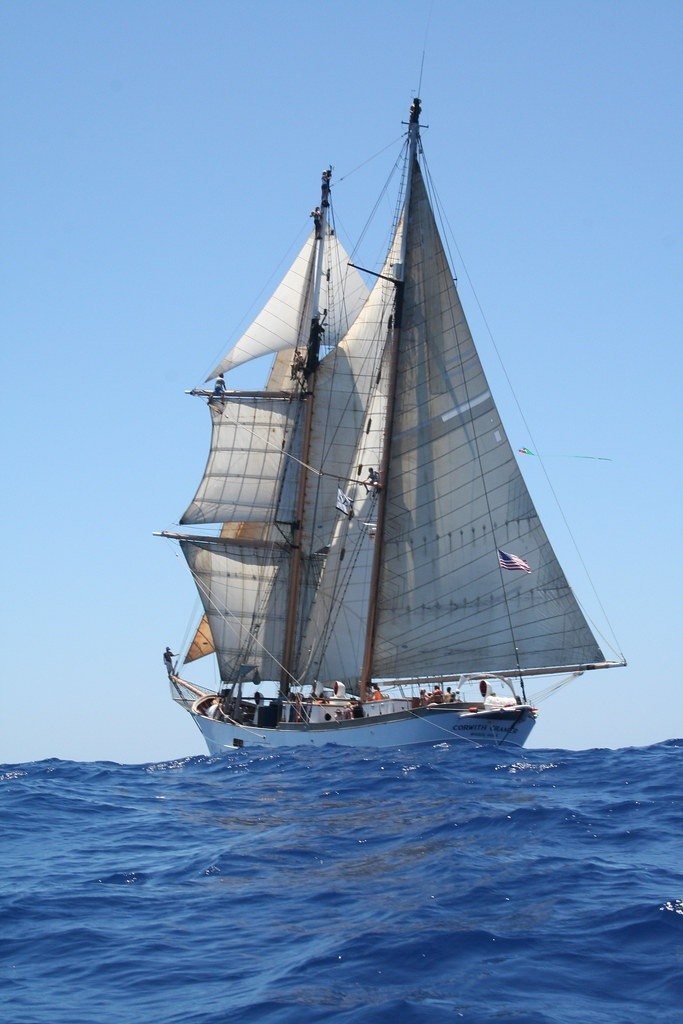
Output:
164 647 180 679
290 350 304 381
419 685 462 706
333 683 382 720
207 373 226 406
359 467 380 497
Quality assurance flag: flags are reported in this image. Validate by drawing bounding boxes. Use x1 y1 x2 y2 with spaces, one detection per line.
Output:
496 548 531 574
335 489 353 516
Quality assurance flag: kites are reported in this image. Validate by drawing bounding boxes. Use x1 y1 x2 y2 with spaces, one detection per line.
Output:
518 448 612 461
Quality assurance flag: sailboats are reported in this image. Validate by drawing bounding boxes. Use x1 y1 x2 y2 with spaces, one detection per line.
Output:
152 50 628 758
